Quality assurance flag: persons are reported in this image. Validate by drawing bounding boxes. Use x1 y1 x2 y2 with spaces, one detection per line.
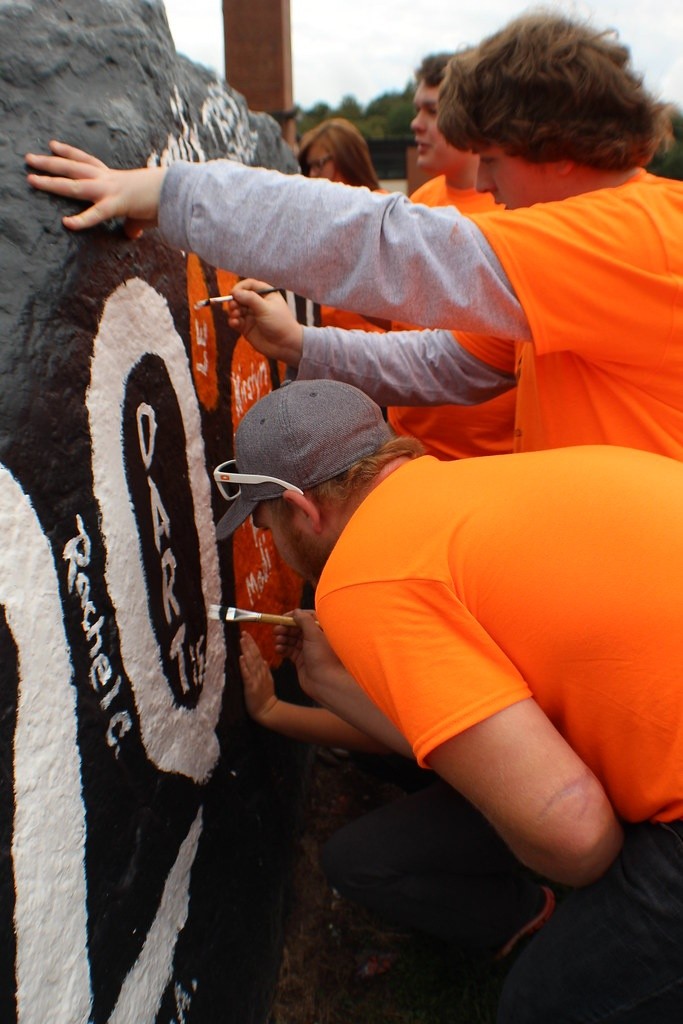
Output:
212 380 683 1024
25 18 683 465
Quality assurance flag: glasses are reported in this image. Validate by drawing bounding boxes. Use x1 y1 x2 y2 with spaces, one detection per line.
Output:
214 459 304 500
305 155 332 176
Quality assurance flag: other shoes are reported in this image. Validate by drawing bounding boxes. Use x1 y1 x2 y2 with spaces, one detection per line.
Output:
450 885 555 969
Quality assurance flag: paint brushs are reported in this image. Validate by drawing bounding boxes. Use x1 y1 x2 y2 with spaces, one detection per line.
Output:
207 604 320 627
193 287 283 309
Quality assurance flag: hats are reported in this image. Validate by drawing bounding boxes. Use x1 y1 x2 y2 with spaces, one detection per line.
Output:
216 379 391 540
